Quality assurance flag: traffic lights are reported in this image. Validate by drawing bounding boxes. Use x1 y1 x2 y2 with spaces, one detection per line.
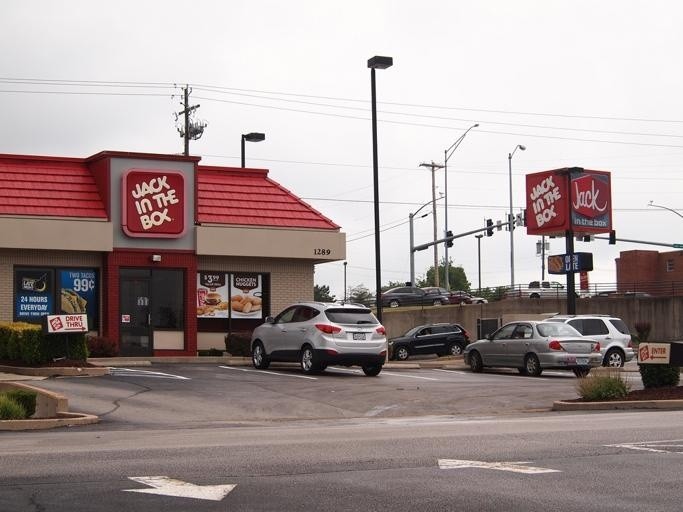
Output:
508 215 514 231
446 230 452 247
609 230 615 244
486 219 493 237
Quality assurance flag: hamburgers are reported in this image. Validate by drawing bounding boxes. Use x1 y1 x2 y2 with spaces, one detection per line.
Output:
205 293 221 305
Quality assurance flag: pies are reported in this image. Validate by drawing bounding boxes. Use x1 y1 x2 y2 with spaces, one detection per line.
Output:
61 288 87 314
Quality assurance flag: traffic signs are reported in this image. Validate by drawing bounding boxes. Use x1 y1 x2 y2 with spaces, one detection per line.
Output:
637 343 670 364
47 314 87 333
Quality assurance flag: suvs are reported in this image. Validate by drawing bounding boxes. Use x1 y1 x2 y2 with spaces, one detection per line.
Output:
388 321 470 361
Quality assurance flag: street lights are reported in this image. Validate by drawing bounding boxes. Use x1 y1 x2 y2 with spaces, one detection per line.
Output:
444 124 479 290
367 56 392 326
536 240 549 280
241 133 264 168
508 144 525 291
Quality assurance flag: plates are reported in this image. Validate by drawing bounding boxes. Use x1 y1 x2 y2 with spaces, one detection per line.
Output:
233 310 257 316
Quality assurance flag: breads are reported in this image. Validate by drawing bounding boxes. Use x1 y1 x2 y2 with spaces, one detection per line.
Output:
232 295 261 313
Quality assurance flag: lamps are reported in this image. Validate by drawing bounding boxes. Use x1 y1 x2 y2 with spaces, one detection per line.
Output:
153 254 162 262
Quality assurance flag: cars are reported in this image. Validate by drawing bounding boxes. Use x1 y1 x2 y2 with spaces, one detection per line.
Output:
528 281 580 298
463 320 603 378
249 302 387 376
542 315 634 368
381 285 488 308
